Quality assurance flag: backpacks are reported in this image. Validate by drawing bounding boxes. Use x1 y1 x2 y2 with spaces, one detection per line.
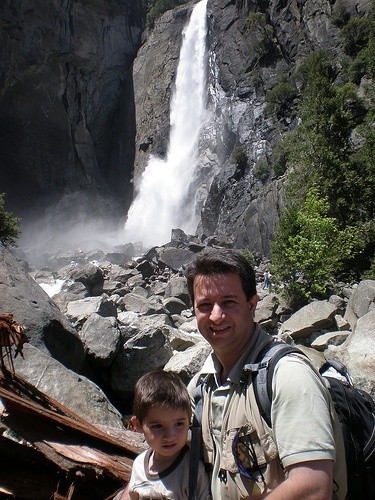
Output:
252 343 374 500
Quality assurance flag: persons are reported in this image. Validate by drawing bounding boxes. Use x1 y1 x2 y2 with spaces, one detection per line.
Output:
117 370 212 500
117 249 341 500
